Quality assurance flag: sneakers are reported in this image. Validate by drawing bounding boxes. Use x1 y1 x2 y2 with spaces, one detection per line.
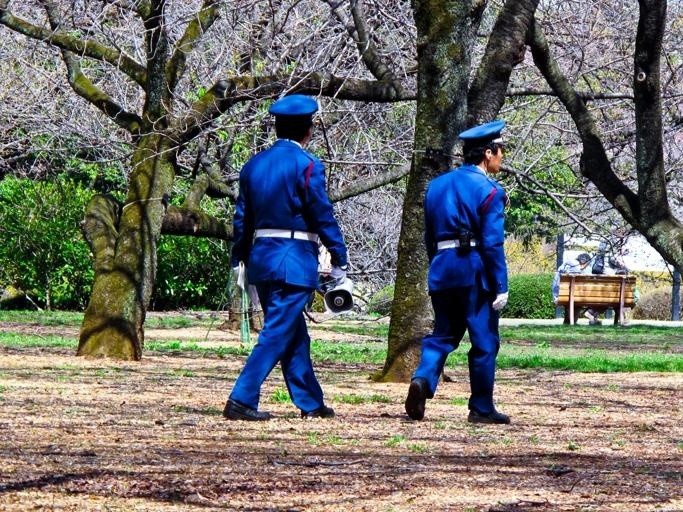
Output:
584 310 595 321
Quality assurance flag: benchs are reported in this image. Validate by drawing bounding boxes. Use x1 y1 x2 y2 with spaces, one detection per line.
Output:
554 273 637 326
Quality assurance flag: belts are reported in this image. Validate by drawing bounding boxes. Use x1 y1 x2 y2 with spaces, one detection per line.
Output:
253 229 320 247
437 239 477 250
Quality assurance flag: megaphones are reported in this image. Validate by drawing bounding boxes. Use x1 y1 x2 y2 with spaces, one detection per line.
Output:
325 278 354 315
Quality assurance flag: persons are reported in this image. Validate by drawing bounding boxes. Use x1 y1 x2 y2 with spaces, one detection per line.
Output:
221 95 348 422
552 254 591 326
405 119 511 424
584 220 629 322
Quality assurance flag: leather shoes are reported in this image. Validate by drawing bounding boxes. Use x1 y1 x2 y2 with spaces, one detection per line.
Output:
222 398 270 421
468 409 510 424
405 377 428 420
301 404 334 419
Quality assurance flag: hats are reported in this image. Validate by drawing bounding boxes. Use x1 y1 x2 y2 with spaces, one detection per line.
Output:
269 94 319 120
459 119 507 147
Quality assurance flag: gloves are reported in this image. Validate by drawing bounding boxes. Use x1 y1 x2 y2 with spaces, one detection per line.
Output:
233 266 240 275
491 291 508 311
329 265 347 286
